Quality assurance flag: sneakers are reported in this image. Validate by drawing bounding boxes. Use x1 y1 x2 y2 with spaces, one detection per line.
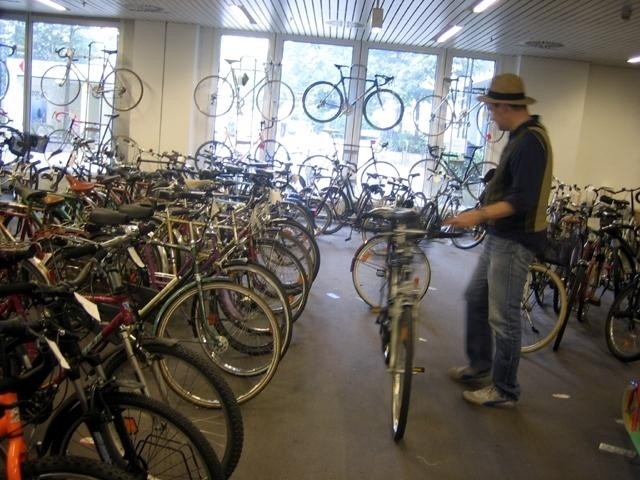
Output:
448 364 490 384
463 384 517 410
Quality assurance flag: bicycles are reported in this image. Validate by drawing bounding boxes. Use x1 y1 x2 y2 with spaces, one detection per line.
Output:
192 56 296 126
302 62 405 131
527 177 638 364
0 109 500 479
0 42 20 100
40 40 144 112
410 74 508 143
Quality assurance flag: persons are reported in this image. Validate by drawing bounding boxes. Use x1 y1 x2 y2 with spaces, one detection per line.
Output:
433 72 555 409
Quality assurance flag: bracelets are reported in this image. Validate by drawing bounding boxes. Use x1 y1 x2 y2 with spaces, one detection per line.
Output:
477 206 492 225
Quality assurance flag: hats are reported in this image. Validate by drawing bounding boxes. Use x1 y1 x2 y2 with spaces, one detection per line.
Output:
477 73 536 106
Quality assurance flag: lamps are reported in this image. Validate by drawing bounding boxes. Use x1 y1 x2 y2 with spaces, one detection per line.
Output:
367 0 385 35
